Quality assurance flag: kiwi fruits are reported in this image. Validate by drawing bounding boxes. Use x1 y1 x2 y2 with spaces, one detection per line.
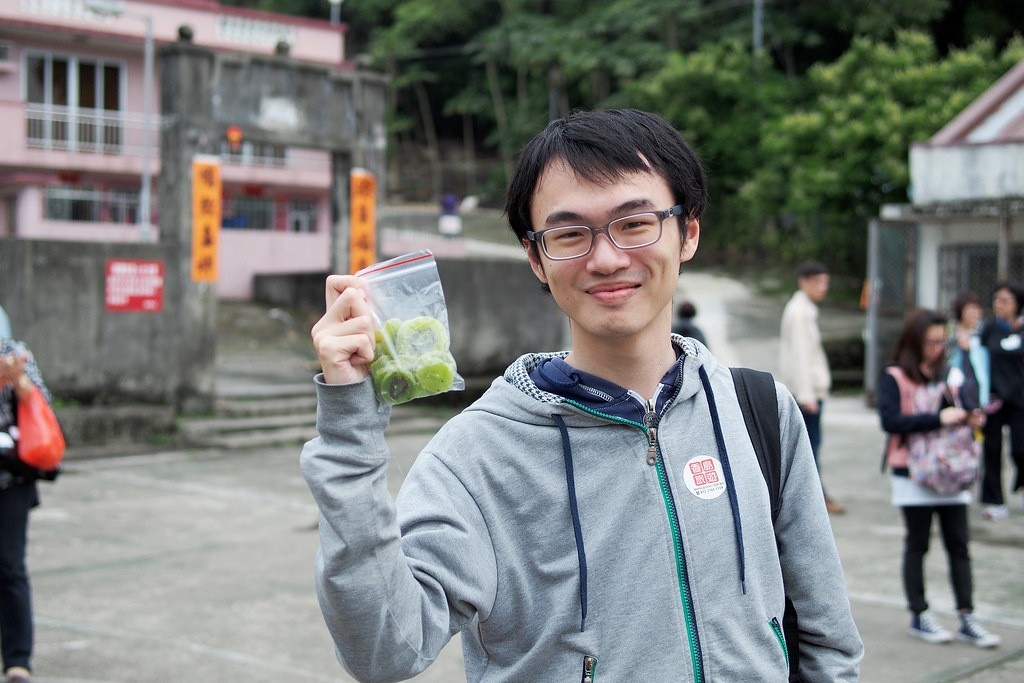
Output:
368 316 453 404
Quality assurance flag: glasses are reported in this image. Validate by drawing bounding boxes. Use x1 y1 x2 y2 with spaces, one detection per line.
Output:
526 203 685 260
927 338 946 347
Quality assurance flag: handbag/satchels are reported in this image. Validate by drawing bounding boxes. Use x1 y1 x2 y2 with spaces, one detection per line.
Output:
19 387 65 471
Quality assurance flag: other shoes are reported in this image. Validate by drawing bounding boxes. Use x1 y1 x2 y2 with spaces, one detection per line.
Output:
824 494 848 514
5 667 31 682
983 504 1010 521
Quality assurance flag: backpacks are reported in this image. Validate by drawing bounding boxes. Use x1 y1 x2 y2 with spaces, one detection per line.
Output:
907 381 983 494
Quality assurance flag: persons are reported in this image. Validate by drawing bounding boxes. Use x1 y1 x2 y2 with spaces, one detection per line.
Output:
776 261 845 518
944 289 992 442
874 307 1005 648
0 304 68 682
671 302 707 347
974 278 1024 522
300 109 865 683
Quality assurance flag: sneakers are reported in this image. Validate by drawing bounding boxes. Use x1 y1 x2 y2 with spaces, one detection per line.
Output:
955 614 1002 648
906 615 955 641
